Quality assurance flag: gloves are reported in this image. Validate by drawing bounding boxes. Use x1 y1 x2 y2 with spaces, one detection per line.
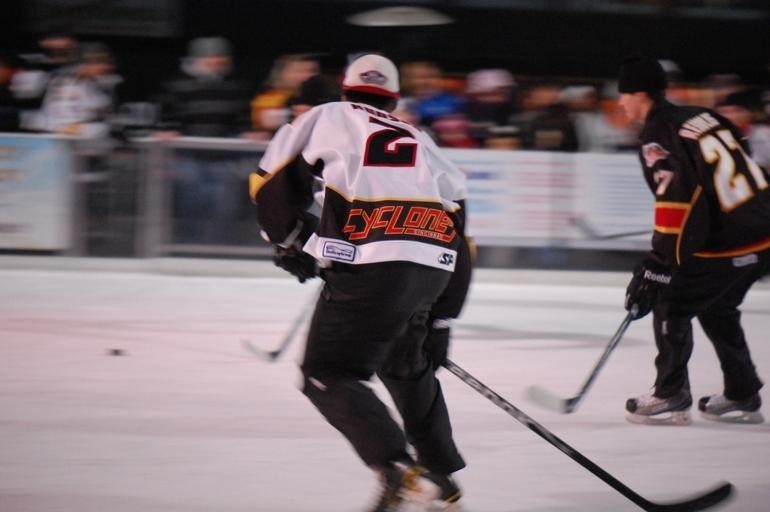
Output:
276 248 316 284
423 317 452 371
624 249 684 320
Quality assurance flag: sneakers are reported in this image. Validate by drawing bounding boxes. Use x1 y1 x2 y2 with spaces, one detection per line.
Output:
698 392 762 415
626 388 693 416
373 451 462 512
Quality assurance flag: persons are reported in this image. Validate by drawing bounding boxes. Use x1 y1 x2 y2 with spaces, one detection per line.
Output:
617 57 770 415
248 51 475 512
1 24 769 250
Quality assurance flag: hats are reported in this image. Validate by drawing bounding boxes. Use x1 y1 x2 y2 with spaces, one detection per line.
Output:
341 54 401 100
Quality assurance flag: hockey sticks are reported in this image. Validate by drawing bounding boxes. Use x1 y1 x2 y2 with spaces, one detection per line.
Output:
239 281 324 361
566 216 652 240
525 310 638 413
440 357 732 511
